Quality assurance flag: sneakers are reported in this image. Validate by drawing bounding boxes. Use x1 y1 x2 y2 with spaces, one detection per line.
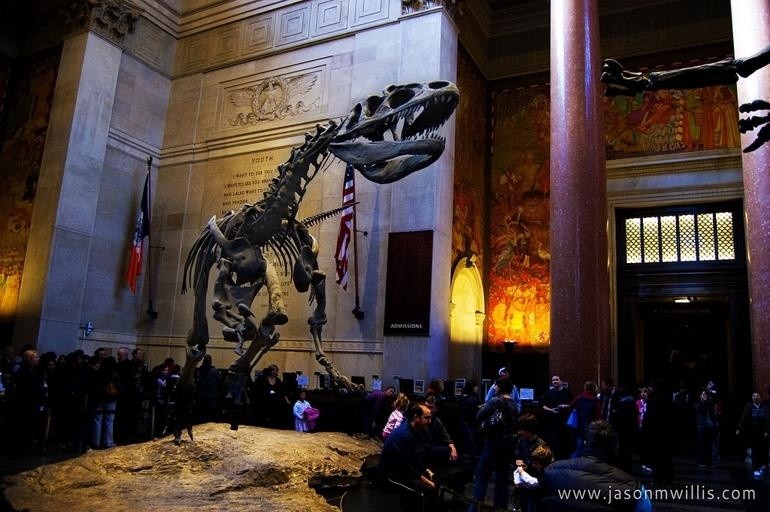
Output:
754 468 764 476
641 464 653 473
697 462 706 468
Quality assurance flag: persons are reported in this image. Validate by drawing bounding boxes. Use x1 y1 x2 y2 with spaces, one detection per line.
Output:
365 362 770 511
260 363 291 426
197 354 221 418
1 346 180 460
292 390 312 433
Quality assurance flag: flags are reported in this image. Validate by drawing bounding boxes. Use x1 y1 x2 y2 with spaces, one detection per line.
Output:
125 173 150 295
334 165 353 291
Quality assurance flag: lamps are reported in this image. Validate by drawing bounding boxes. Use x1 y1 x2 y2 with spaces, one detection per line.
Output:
499 341 518 351
466 252 478 268
